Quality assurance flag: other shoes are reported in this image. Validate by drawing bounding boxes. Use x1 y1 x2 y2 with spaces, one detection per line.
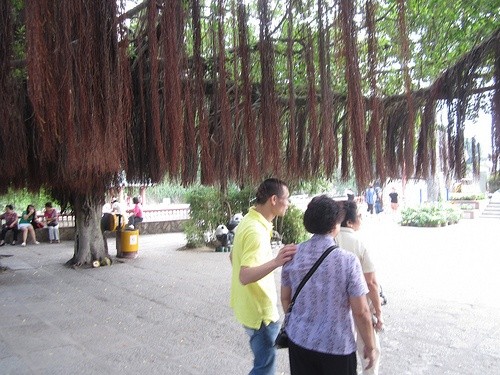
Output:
129 224 134 230
12 241 15 246
34 241 40 245
0 243 5 246
21 242 26 246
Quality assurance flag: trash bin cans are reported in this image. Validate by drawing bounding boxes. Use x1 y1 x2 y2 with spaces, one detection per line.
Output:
116 229 139 258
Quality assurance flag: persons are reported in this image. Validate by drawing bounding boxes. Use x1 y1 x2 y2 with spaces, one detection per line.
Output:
348 184 401 223
336 197 384 375
228 178 297 375
280 194 378 375
0 192 144 247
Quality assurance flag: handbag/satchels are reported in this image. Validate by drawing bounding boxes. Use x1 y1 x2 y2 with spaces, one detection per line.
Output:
31 222 44 228
47 221 58 226
275 329 290 349
